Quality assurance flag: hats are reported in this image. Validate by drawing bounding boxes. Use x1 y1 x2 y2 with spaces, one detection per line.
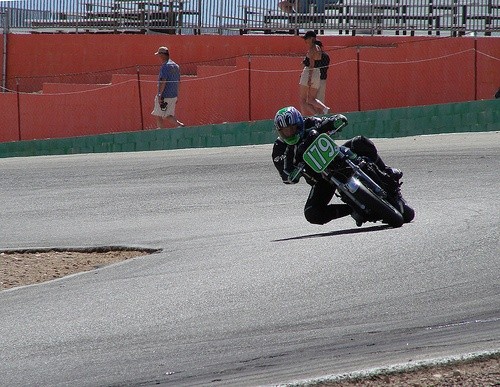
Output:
303 31 316 40
154 46 168 55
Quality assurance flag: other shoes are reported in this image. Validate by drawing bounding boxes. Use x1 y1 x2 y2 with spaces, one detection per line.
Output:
177 122 184 128
351 211 362 227
384 166 403 180
322 106 331 115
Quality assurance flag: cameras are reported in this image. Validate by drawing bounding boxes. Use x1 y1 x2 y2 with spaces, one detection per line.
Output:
160 99 168 111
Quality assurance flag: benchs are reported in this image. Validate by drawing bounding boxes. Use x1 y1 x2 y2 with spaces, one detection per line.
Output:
23 0 500 36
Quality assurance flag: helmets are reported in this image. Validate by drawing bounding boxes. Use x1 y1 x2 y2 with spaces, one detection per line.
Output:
274 107 304 146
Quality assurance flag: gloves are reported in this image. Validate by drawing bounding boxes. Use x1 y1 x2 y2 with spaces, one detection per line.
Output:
333 116 348 132
288 169 303 183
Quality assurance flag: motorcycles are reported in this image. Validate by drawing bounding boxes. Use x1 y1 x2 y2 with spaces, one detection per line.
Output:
272 98 406 228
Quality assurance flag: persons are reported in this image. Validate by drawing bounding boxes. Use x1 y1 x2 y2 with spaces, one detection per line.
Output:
152 47 184 129
279 0 338 23
299 31 331 115
272 107 402 225
